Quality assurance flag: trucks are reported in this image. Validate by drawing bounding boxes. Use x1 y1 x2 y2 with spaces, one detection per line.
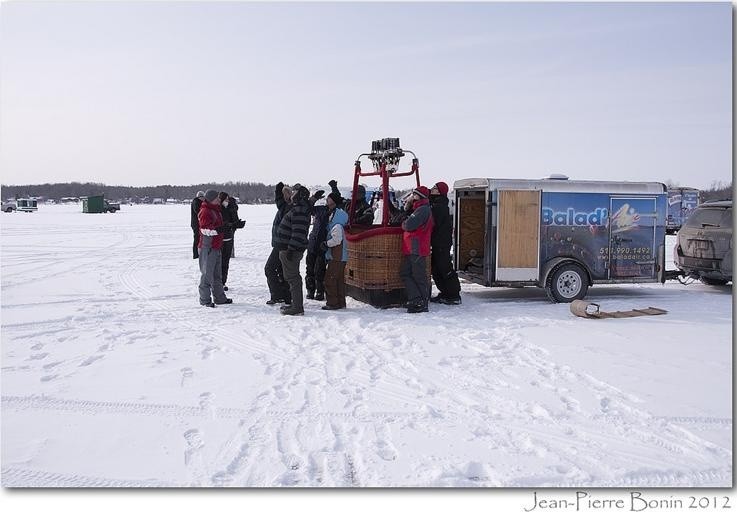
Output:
1 197 38 213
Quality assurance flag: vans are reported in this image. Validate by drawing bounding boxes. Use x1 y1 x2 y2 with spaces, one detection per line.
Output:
674 198 734 287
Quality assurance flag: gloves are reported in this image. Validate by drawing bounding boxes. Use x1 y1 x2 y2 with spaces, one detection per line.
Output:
275 182 284 193
285 245 297 261
312 190 325 200
215 222 233 234
230 219 246 229
328 179 339 189
318 241 328 253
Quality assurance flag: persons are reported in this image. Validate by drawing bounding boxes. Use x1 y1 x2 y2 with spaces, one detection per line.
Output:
229 197 238 258
219 192 245 291
266 180 462 316
197 189 232 307
191 191 204 259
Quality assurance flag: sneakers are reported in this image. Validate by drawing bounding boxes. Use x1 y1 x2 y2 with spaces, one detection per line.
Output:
407 301 429 314
280 304 305 316
223 284 229 291
429 292 463 305
266 298 291 305
305 290 315 300
315 291 325 300
322 303 347 311
193 255 199 259
200 296 233 309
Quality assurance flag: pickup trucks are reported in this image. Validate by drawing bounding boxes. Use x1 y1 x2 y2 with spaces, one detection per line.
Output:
103 200 121 213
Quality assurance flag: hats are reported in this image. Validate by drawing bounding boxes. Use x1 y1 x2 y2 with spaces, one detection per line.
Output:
203 188 218 203
436 181 449 197
412 185 430 199
329 192 341 204
290 182 310 202
